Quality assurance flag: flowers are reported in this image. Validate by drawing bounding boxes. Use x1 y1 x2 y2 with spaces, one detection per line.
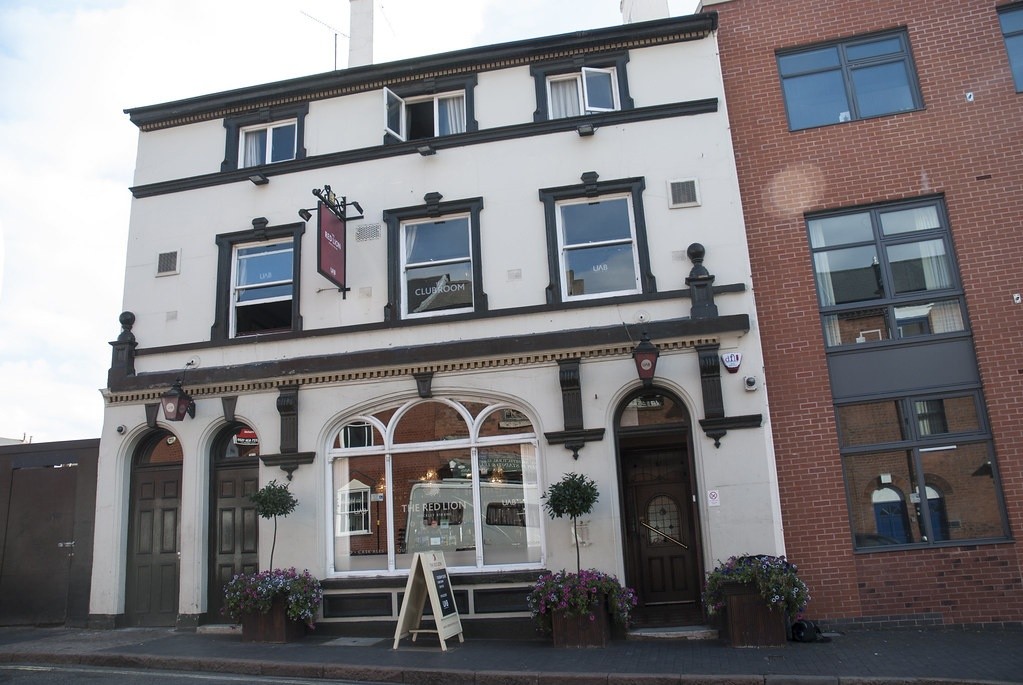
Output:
219 566 326 630
526 569 637 633
700 553 813 624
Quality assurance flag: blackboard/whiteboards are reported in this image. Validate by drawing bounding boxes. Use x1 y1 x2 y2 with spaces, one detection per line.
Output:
431 567 458 620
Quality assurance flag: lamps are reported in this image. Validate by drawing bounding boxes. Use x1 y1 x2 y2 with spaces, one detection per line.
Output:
161 375 196 422
632 329 660 388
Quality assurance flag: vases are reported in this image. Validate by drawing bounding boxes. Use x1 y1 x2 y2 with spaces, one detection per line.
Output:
551 607 613 648
723 582 787 646
241 605 308 643
